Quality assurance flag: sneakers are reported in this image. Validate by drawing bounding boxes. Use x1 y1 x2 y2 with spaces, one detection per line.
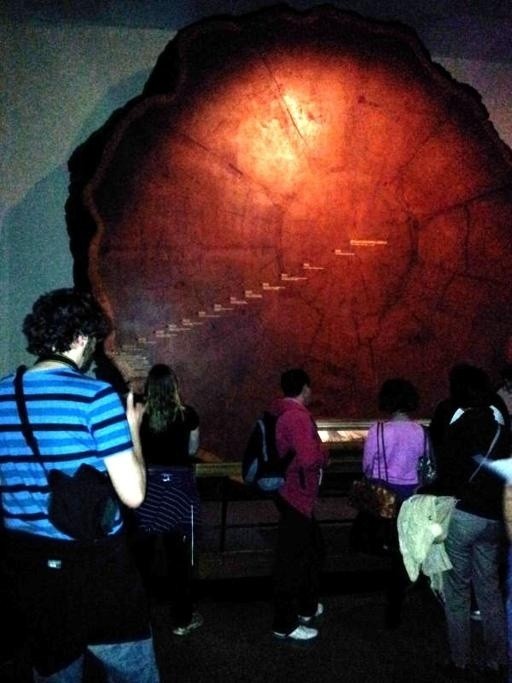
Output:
470 610 481 621
472 661 510 683
172 611 204 635
440 663 474 683
296 601 323 622
272 621 319 640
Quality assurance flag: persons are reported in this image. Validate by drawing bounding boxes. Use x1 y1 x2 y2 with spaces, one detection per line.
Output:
362 377 435 627
1 287 163 680
425 362 511 683
263 367 333 640
129 365 206 635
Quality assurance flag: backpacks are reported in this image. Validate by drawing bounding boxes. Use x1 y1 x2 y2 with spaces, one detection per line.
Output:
241 407 296 492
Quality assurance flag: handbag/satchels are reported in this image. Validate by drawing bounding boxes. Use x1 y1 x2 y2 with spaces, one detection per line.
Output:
417 456 438 494
46 464 115 542
348 476 401 521
412 494 460 543
